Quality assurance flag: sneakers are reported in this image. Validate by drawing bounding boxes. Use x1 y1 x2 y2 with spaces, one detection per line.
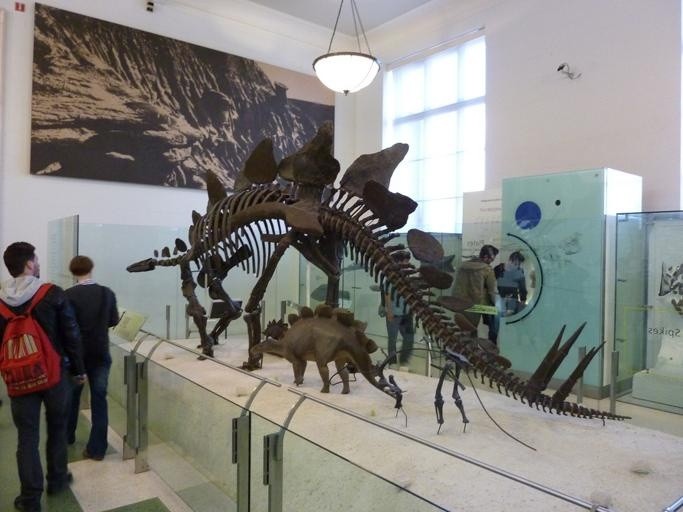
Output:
388 362 416 373
83 449 104 461
45 471 72 497
14 494 42 512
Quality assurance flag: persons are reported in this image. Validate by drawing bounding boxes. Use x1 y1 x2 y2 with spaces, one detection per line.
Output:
449 243 501 375
381 249 417 367
0 241 88 512
491 251 527 316
60 253 118 461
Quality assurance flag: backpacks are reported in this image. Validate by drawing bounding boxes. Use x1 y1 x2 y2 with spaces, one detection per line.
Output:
0 282 61 399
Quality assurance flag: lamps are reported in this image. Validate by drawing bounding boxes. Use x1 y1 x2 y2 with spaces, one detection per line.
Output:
312 0 381 97
